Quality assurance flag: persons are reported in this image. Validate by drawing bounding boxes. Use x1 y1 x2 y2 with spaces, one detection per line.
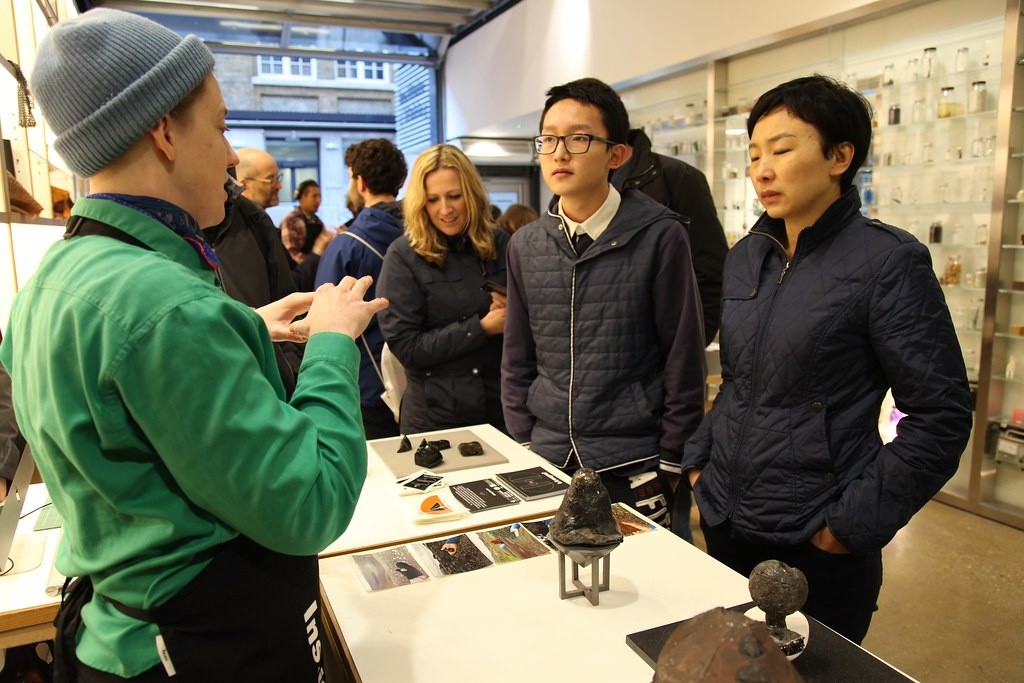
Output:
281 139 409 441
211 147 312 380
682 77 973 645
375 143 510 434
490 203 538 232
500 77 729 545
0 5 389 682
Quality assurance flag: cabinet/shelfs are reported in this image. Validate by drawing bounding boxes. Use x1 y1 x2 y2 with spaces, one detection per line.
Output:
627 0 1024 531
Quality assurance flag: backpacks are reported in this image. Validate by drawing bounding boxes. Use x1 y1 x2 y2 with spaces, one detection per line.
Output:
340 231 408 423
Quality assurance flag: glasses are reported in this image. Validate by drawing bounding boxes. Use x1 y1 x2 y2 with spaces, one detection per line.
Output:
533 134 618 155
244 173 283 188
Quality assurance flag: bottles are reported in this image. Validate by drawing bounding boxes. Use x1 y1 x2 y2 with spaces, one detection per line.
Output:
921 47 937 78
655 98 765 251
888 183 987 203
889 102 900 125
983 135 995 155
967 81 986 114
907 59 919 82
946 296 985 331
938 253 986 289
870 111 877 128
965 349 975 380
956 47 969 72
909 221 919 241
936 87 957 118
929 220 942 244
970 137 984 158
882 64 893 86
952 223 963 244
882 142 962 167
975 224 988 245
912 97 925 122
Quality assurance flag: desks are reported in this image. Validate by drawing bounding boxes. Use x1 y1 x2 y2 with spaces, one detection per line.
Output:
315 422 921 683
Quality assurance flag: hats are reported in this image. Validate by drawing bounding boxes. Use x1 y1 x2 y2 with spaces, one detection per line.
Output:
29 7 215 178
